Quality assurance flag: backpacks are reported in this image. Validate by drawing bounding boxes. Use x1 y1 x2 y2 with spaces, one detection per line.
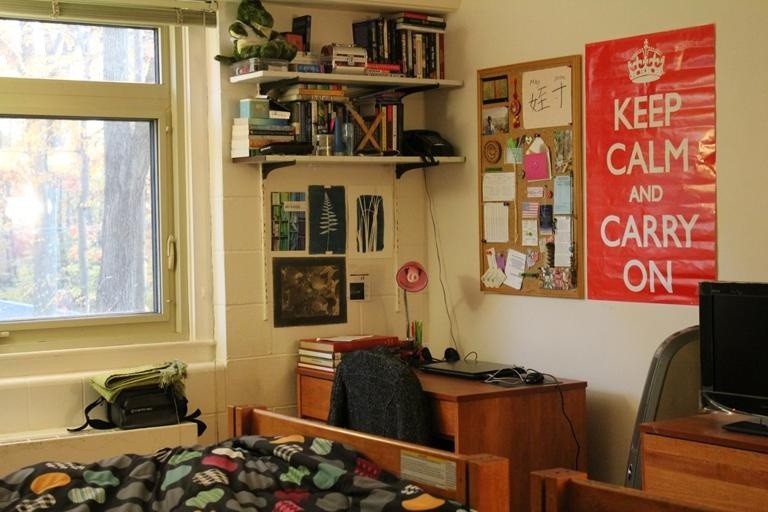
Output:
66 384 206 436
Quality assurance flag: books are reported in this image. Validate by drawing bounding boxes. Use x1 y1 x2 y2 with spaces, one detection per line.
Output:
294 331 400 373
229 10 448 161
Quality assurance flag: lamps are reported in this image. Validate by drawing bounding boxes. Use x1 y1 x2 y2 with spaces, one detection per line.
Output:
396 261 429 339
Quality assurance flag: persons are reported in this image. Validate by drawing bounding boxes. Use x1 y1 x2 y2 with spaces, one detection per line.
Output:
280 267 336 317
483 116 497 135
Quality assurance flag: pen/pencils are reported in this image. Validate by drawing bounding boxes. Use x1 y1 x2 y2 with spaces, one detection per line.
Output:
346 107 349 124
328 102 332 134
411 319 422 345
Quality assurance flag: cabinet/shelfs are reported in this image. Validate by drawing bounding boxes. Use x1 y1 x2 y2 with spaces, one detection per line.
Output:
230 70 466 165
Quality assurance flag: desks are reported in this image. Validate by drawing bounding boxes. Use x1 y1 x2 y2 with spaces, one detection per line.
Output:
293 366 588 511
638 411 767 512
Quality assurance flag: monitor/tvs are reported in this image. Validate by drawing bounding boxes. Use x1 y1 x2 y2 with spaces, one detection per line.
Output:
698 282 768 436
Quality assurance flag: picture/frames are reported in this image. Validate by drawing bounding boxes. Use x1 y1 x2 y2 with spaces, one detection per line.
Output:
477 55 585 299
272 256 347 327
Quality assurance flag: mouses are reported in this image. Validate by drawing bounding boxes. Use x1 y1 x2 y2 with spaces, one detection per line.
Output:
525 373 544 384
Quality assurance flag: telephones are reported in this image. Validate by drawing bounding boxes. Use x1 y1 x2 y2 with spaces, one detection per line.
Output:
402 129 455 158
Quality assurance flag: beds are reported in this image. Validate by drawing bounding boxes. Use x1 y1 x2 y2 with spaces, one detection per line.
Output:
0 404 511 510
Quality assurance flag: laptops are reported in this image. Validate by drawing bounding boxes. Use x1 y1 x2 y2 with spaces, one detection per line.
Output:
418 361 516 381
